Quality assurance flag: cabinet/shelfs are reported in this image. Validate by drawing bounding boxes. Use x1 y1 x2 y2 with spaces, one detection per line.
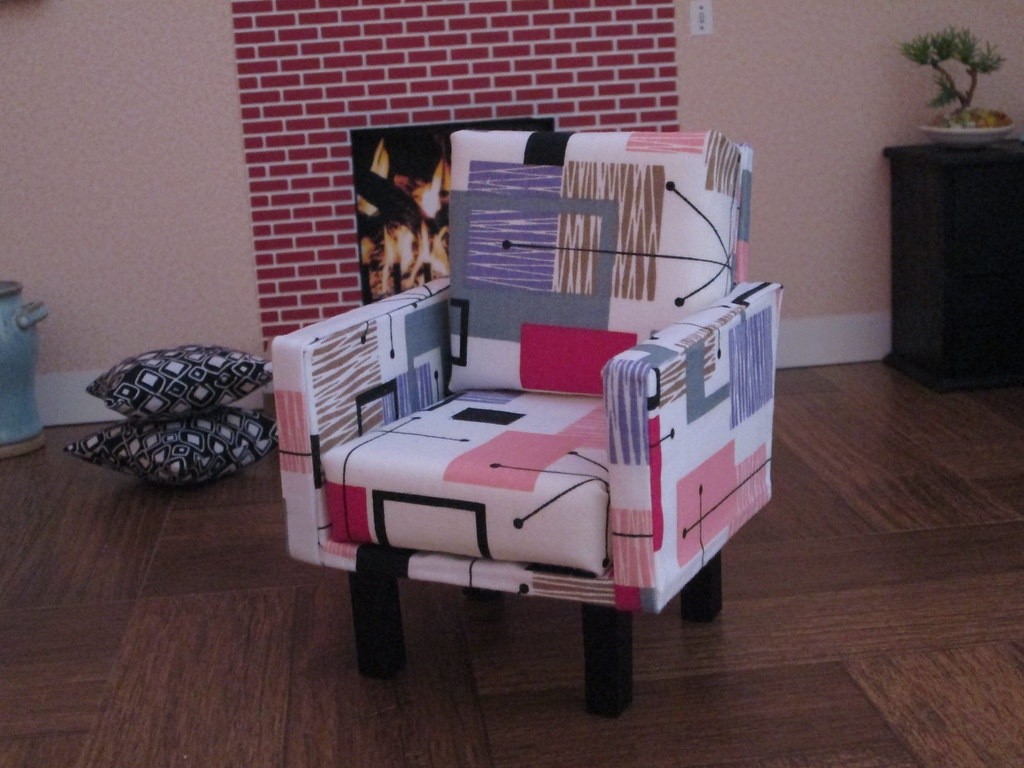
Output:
882 138 1024 392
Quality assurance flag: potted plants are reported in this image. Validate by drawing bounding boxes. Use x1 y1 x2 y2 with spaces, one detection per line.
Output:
894 25 1016 148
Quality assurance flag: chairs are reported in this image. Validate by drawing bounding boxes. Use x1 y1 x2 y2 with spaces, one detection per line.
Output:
270 127 784 711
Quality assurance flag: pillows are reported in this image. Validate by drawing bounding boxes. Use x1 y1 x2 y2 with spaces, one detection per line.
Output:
84 342 278 419
66 407 279 491
